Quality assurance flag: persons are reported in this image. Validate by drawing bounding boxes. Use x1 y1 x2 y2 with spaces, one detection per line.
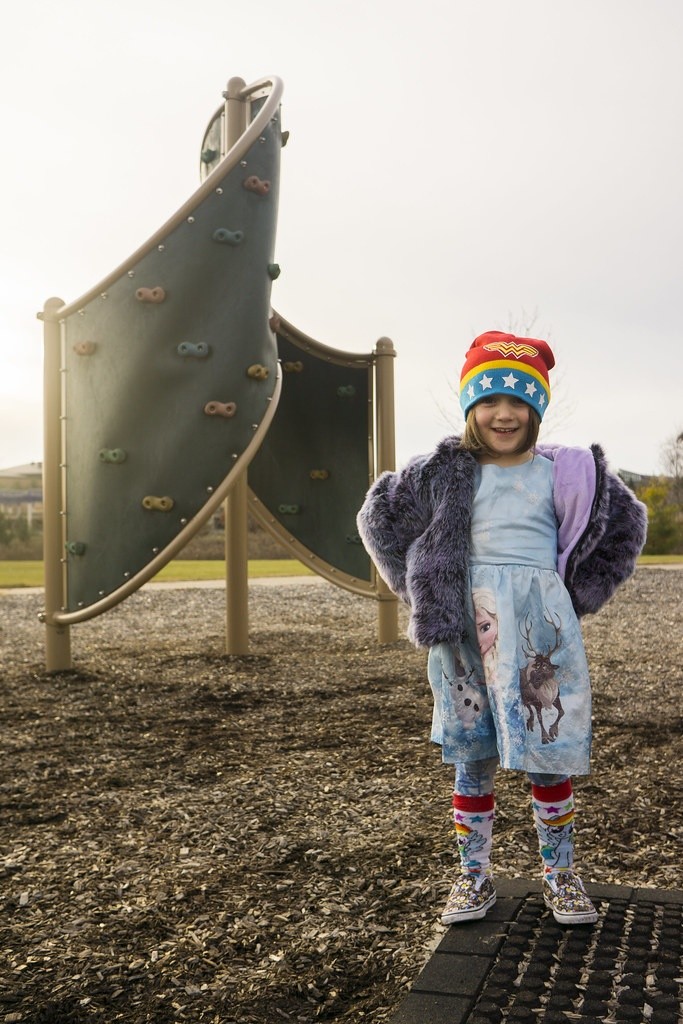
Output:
357 330 649 928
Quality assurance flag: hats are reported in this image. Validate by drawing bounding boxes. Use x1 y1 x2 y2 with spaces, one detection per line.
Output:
459 330 557 421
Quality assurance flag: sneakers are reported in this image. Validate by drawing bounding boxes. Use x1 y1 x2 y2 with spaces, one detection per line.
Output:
440 875 496 924
542 870 599 927
